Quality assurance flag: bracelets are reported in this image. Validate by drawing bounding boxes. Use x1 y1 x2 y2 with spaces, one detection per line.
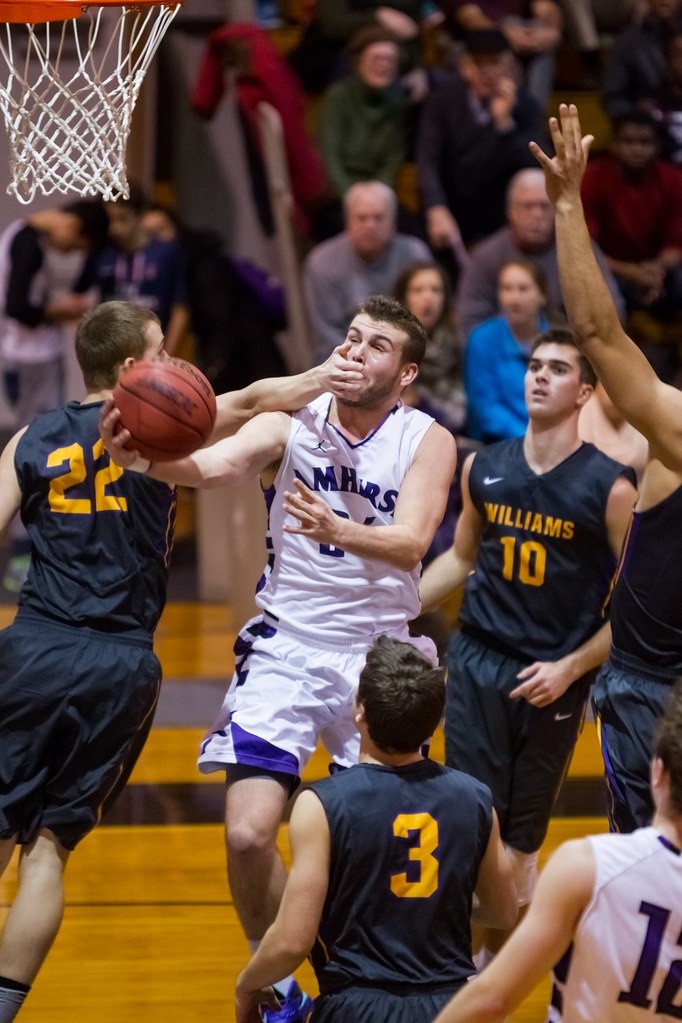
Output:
124 450 152 474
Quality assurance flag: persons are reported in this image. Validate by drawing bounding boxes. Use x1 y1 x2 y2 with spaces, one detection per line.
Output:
529 102 682 482
410 327 636 966
232 635 519 1023
588 450 682 831
0 301 362 1023
0 1 682 606
431 679 682 1023
98 297 458 1021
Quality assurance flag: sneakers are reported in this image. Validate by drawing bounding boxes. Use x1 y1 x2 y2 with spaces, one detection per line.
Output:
260 980 315 1023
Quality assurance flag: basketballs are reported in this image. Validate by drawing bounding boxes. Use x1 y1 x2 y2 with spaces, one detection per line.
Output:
111 357 217 460
